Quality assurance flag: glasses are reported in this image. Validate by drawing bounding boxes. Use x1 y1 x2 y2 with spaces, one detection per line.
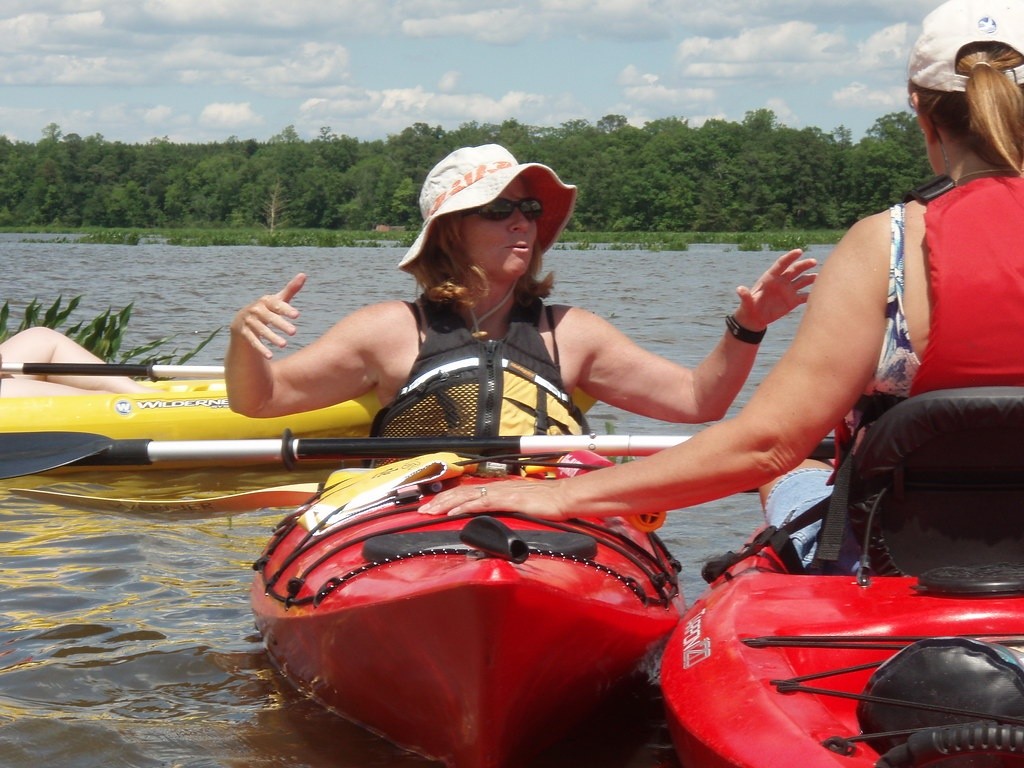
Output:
459 197 545 223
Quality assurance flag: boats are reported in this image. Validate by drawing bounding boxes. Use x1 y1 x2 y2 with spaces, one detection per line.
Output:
247 448 688 767
659 389 1024 768
0 379 601 474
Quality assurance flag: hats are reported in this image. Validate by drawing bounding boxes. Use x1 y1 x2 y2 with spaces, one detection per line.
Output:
396 144 578 279
907 0 1024 92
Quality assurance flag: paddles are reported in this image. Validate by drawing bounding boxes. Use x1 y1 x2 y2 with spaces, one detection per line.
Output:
0 434 835 479
0 363 224 378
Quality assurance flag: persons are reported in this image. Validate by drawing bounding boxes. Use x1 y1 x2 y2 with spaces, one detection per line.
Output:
0 327 167 395
417 1 1024 573
224 140 818 458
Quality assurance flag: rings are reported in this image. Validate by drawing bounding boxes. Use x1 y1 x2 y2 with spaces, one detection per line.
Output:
480 488 486 495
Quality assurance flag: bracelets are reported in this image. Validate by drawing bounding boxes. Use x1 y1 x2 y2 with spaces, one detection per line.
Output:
723 315 768 345
